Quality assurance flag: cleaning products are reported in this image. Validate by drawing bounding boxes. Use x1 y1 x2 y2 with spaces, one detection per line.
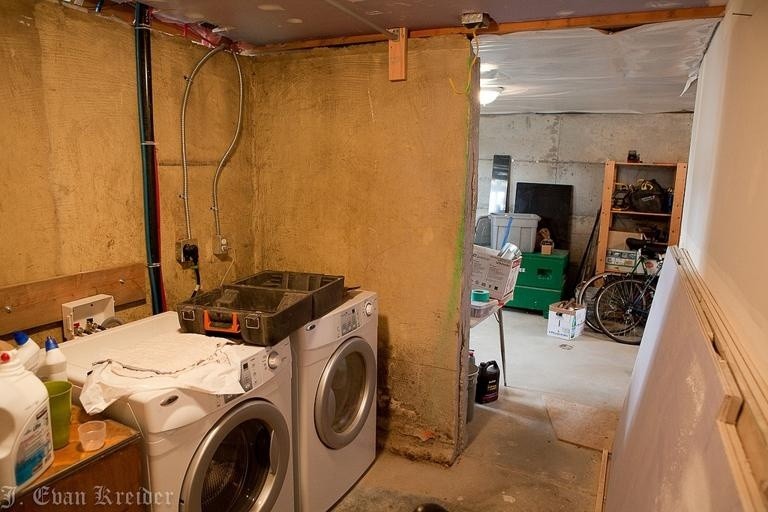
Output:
13 331 42 376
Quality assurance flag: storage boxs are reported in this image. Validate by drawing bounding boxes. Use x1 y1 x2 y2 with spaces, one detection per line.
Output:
472 244 522 301
175 271 345 347
547 301 587 341
488 213 541 253
636 249 660 276
606 231 647 273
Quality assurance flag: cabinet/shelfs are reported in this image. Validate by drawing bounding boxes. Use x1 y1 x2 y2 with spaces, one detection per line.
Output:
503 249 569 319
594 160 688 288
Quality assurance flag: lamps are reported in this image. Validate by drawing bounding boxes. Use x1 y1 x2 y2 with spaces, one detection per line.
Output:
480 88 502 105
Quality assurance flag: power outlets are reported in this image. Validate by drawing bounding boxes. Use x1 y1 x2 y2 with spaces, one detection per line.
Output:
214 235 228 254
175 239 198 262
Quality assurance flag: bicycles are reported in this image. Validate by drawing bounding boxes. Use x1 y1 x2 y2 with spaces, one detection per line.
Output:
589 239 669 346
577 236 667 335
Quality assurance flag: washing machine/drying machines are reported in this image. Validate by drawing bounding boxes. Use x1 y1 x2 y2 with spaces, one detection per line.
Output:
39 310 299 512
289 290 379 512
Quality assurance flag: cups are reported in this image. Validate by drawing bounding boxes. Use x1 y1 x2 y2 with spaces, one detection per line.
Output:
43 380 107 452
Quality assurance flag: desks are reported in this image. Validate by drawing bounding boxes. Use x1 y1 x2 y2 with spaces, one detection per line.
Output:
470 299 506 386
0 405 144 512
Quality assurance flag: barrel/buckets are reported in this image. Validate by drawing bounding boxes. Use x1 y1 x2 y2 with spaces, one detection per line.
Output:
1 349 53 501
475 360 500 404
575 281 600 330
